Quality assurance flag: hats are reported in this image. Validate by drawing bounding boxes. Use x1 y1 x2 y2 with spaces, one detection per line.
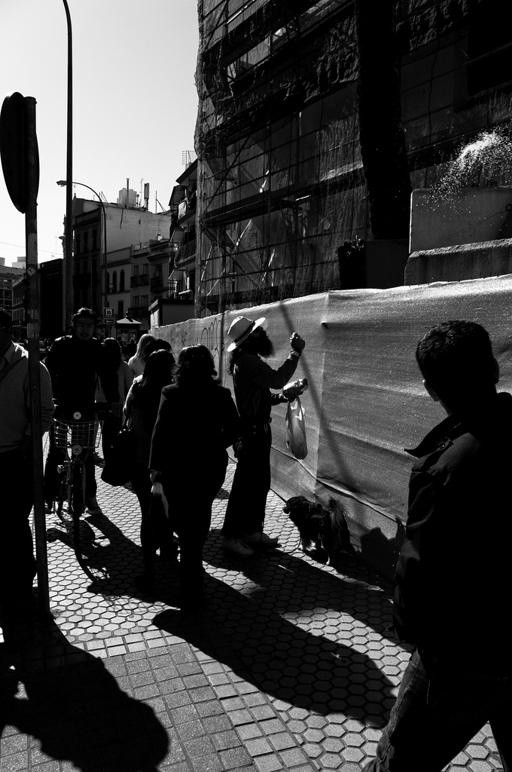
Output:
227 316 266 352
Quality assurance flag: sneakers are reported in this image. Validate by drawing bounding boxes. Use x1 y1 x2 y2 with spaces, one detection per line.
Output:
225 534 278 557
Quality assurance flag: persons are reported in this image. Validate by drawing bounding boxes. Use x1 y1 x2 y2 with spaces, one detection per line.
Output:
359 317 511 770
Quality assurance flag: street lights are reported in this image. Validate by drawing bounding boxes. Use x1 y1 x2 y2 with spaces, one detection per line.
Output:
56 179 108 308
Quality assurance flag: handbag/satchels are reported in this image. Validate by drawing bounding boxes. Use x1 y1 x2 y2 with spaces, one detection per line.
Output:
286 397 307 459
101 427 129 487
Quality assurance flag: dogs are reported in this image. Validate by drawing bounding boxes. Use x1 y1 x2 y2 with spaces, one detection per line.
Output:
281 495 351 566
358 515 407 593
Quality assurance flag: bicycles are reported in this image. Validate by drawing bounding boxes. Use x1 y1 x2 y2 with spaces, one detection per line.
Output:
44 398 110 544
